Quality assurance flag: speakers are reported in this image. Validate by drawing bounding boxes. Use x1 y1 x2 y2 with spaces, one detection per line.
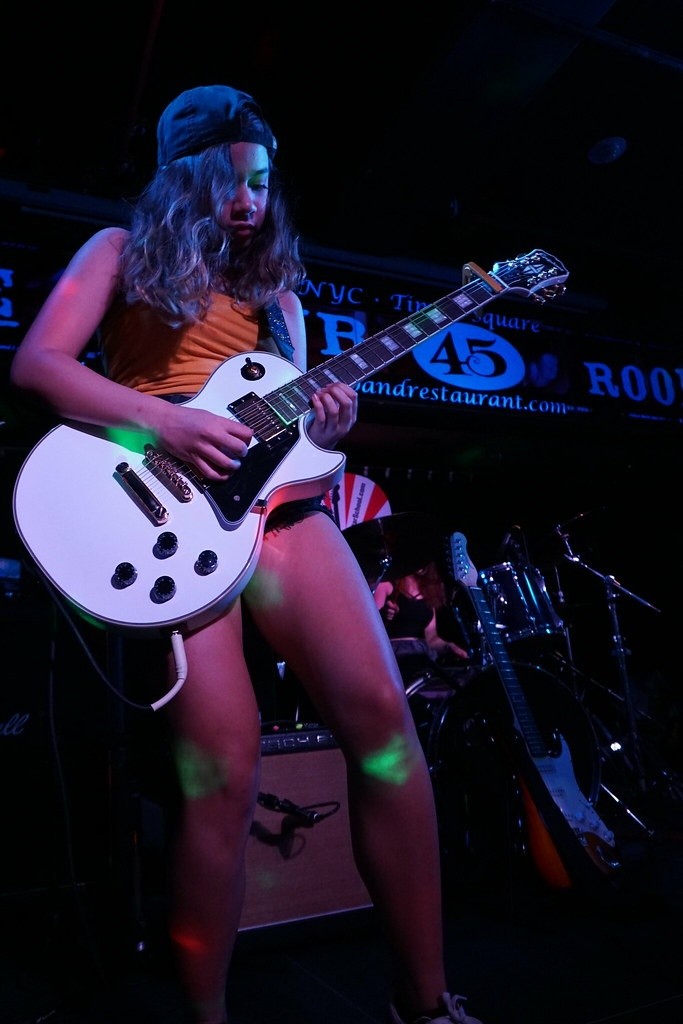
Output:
134 728 376 934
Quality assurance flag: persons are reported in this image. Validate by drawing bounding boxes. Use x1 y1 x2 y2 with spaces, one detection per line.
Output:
373 563 469 658
10 85 484 1024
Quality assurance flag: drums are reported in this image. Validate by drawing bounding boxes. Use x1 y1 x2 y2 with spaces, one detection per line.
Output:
404 659 603 898
451 560 568 657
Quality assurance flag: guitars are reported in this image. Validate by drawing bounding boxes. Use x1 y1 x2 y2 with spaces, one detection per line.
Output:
11 248 571 640
444 532 624 893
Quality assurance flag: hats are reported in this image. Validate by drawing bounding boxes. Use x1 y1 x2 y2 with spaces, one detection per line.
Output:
157 85 276 166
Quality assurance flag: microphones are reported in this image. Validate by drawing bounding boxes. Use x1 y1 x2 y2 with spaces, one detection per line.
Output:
279 798 320 823
501 525 516 546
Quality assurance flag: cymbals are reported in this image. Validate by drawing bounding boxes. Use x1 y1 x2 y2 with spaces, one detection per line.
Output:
529 501 610 554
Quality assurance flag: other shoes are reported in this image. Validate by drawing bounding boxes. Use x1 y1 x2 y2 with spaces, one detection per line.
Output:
388 991 480 1024
